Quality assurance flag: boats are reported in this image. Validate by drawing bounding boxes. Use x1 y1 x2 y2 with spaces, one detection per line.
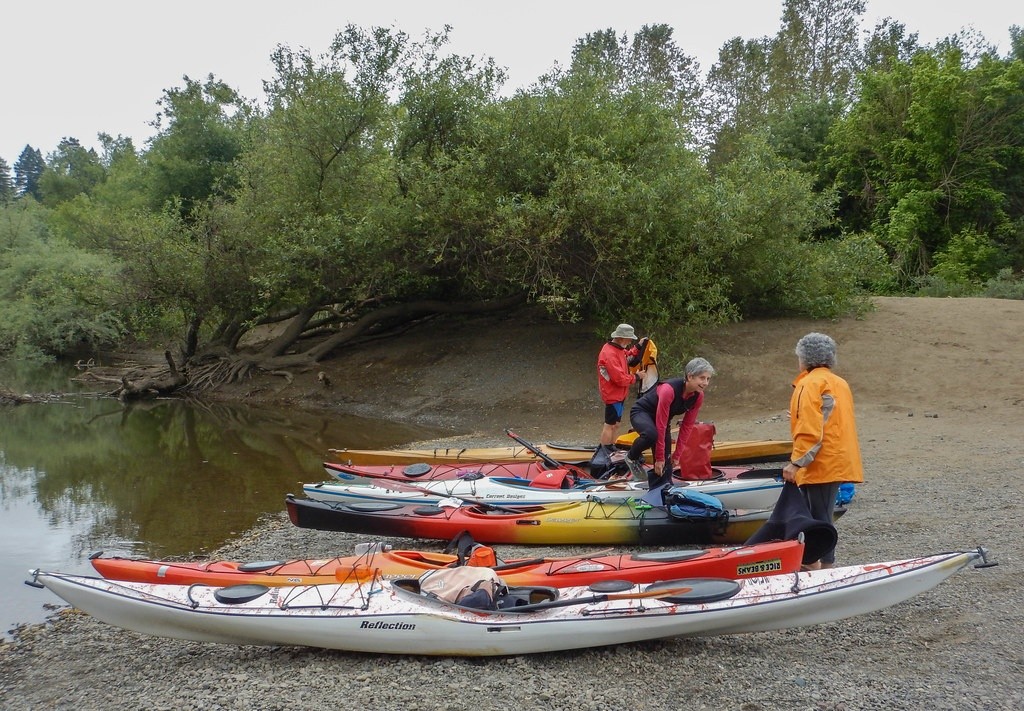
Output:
26 547 990 658
285 493 848 546
327 428 794 467
322 461 784 484
303 477 787 513
88 532 805 592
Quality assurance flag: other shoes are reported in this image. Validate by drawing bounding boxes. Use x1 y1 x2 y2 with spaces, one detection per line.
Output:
603 444 620 454
802 559 833 571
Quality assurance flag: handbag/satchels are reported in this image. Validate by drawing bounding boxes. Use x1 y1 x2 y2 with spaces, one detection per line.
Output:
419 564 509 610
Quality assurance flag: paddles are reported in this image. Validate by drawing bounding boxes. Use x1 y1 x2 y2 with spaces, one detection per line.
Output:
497 587 693 612
489 548 615 571
370 478 526 514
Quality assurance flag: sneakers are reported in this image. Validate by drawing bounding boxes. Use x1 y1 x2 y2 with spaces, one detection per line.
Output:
624 452 647 479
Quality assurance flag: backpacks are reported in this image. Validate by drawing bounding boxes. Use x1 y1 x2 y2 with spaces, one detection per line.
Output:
659 483 728 537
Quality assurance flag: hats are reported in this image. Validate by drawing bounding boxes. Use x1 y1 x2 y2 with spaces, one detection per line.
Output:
610 323 638 340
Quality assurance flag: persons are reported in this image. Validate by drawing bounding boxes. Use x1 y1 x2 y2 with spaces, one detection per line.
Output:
597 323 650 453
624 356 714 480
782 332 864 572
